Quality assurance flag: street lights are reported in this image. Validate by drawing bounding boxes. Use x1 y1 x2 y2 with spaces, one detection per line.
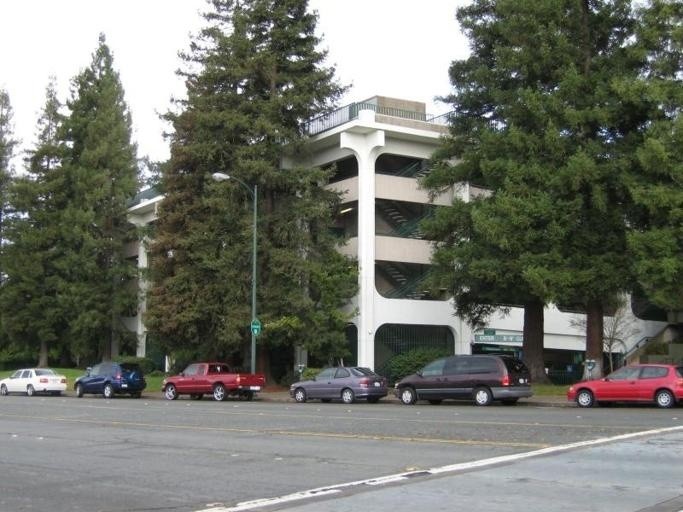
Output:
211 171 258 373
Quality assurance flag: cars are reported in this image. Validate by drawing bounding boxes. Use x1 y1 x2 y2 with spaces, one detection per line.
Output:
566 363 682 408
290 368 385 403
0 368 68 397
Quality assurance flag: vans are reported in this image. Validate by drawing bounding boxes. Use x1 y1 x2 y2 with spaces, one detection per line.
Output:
394 353 532 405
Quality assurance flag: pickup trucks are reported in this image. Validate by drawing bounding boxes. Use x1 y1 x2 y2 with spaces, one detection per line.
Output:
161 362 265 403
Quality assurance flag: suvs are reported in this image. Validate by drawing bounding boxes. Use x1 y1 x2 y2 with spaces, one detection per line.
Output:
74 362 147 400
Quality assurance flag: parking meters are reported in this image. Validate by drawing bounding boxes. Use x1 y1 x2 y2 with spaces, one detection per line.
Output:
298 364 305 380
586 358 595 380
